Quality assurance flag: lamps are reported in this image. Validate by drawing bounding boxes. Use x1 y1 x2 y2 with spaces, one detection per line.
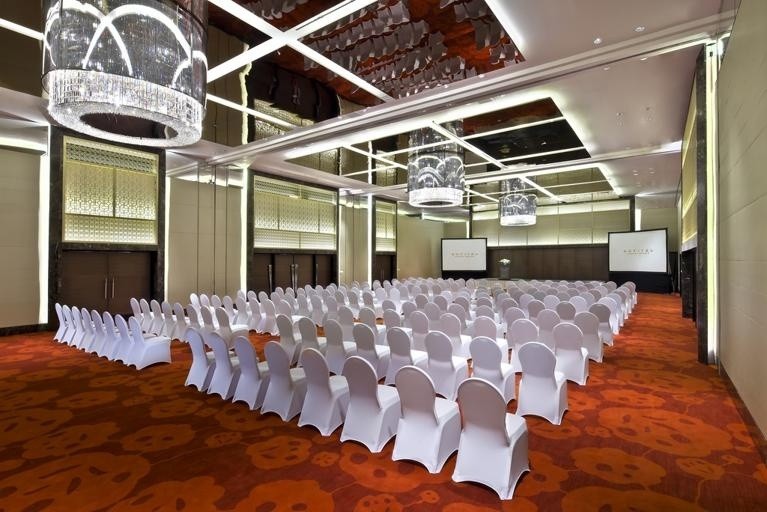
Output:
498 163 539 227
406 118 467 209
41 0 210 148
